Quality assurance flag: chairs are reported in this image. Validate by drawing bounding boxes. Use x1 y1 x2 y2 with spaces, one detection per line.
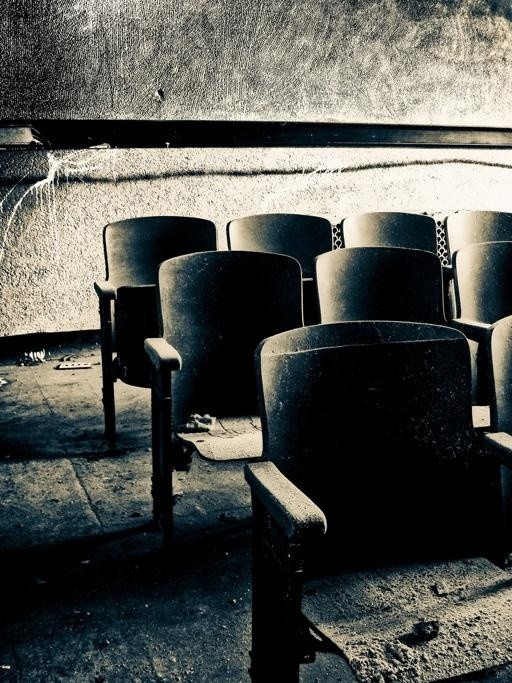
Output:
340 212 453 287
442 211 511 252
451 241 512 406
315 245 446 324
96 215 218 443
242 319 511 682
226 214 333 303
144 250 304 531
489 315 510 506
